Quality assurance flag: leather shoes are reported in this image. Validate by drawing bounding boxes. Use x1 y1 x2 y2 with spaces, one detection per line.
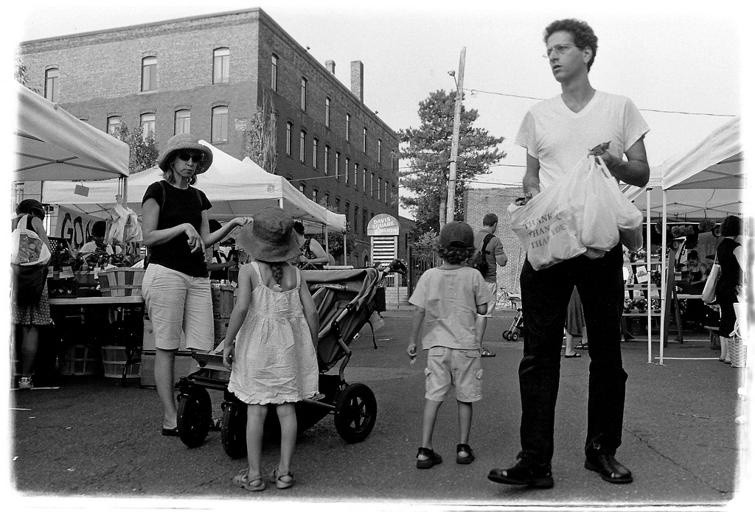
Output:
584 451 633 483
488 459 553 488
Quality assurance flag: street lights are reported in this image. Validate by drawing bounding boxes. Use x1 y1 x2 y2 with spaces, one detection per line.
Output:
446 70 466 225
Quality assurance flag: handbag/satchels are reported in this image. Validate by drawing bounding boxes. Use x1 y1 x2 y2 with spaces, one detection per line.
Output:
9 214 51 265
470 250 489 276
702 264 721 304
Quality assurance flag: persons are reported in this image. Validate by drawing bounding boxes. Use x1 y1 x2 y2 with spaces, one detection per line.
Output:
487 18 652 489
11 199 53 388
291 221 330 270
466 213 507 357
564 285 586 357
683 248 706 285
223 206 319 491
407 220 490 469
374 263 387 318
139 133 254 436
716 214 743 364
79 221 105 253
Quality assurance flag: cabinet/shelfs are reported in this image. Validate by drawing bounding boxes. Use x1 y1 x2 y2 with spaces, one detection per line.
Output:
623 248 687 350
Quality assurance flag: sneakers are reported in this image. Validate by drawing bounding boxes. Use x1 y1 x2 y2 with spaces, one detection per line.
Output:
19 376 34 389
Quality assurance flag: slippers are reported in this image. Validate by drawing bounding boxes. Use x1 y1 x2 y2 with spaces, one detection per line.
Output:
162 426 178 435
565 352 581 358
210 418 222 431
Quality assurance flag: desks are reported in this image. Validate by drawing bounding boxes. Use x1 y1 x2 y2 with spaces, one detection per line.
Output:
49 296 143 378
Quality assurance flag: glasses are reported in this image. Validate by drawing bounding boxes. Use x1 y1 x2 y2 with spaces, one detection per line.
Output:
179 153 200 162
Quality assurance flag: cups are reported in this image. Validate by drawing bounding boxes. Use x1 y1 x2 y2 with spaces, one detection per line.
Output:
617 211 644 252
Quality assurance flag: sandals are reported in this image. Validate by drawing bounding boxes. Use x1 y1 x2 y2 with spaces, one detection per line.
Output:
456 444 474 462
268 470 294 489
233 470 265 491
481 347 496 357
575 343 588 349
416 448 441 469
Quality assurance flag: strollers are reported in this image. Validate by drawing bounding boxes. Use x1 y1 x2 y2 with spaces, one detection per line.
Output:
501 287 529 341
175 265 401 447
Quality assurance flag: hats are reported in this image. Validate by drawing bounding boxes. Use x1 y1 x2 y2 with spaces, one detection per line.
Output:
235 206 307 262
441 221 474 249
156 134 213 174
